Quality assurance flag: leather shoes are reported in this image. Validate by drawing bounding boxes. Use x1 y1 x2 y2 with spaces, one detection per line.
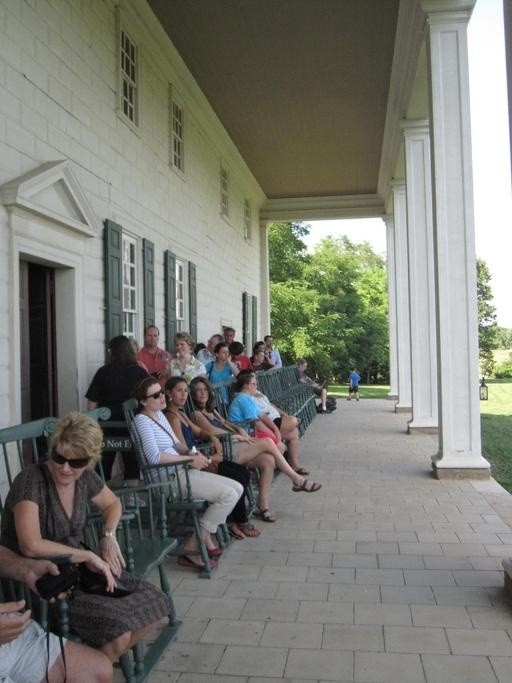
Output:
205 546 224 555
320 409 332 414
178 555 218 569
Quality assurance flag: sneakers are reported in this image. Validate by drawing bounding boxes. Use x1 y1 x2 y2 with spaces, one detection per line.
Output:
228 522 262 539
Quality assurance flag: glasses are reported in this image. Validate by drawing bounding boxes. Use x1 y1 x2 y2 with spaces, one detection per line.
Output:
50 443 93 469
145 388 165 400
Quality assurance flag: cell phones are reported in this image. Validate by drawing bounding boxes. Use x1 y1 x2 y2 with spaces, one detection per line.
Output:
34 559 80 601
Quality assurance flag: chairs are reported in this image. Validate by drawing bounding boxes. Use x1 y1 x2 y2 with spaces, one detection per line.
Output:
0 406 184 682
122 365 317 579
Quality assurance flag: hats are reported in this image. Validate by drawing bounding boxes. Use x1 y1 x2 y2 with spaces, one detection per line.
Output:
229 342 243 356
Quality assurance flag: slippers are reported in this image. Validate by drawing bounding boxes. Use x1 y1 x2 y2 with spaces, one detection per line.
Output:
295 468 310 475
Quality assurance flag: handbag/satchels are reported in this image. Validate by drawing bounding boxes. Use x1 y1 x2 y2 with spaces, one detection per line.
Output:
79 562 132 599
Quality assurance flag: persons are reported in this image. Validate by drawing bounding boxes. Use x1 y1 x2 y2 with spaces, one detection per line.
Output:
347 366 361 401
0 542 114 683
0 411 171 664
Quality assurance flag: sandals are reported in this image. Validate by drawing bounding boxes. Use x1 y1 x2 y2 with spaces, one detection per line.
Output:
252 504 276 523
292 479 322 493
